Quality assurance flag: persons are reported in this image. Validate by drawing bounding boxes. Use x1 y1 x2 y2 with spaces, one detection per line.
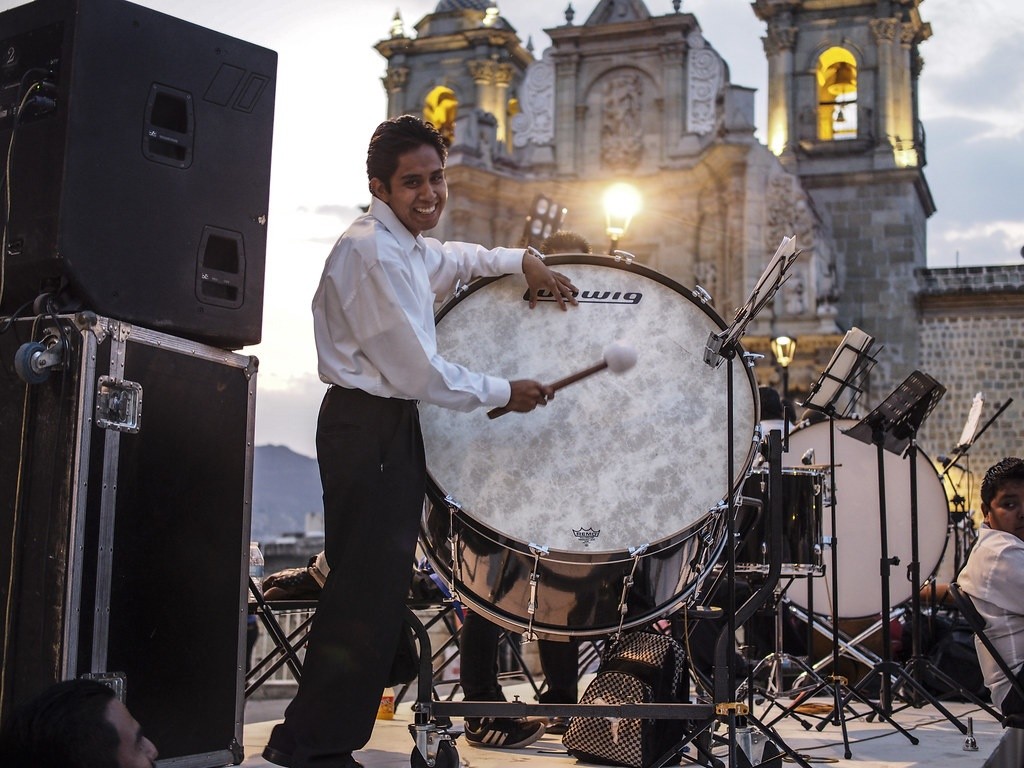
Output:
460 229 591 749
0 680 159 768
262 116 579 768
957 457 1024 719
753 387 829 458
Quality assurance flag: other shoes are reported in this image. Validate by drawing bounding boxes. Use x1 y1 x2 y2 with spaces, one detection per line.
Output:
262 724 364 768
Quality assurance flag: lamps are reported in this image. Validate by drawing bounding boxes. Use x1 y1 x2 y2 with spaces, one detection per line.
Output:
771 334 796 368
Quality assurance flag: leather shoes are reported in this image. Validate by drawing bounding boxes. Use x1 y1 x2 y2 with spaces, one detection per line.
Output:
546 716 570 733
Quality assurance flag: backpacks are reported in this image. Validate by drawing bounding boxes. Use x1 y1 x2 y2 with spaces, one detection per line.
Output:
562 630 689 768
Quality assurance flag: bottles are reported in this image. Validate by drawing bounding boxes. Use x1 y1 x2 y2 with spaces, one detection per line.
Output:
376 687 395 720
248 542 264 603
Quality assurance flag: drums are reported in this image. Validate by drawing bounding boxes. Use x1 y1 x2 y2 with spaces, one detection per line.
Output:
779 414 960 619
710 462 830 579
932 515 979 587
399 249 767 649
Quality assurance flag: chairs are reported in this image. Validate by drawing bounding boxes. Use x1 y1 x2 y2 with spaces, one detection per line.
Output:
245 574 432 716
947 583 1024 730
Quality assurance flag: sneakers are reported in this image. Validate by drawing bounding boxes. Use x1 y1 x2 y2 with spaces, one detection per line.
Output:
464 714 548 749
